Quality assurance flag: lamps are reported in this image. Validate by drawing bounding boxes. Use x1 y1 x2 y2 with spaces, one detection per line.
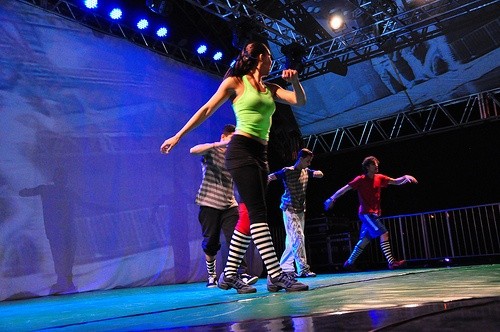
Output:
328 62 348 76
282 44 304 74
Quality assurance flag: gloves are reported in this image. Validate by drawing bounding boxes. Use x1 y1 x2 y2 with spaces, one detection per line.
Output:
324 197 335 211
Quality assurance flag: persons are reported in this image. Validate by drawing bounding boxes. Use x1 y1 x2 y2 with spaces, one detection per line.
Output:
268 148 323 277
160 44 308 295
189 124 259 288
324 157 418 273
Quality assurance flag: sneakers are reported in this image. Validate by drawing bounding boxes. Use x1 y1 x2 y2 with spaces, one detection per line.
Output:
267 273 309 292
241 273 258 285
218 272 257 294
206 275 218 287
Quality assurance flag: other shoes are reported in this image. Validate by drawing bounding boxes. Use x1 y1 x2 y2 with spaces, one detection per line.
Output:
343 261 357 271
304 271 316 277
286 272 298 278
389 260 405 269
